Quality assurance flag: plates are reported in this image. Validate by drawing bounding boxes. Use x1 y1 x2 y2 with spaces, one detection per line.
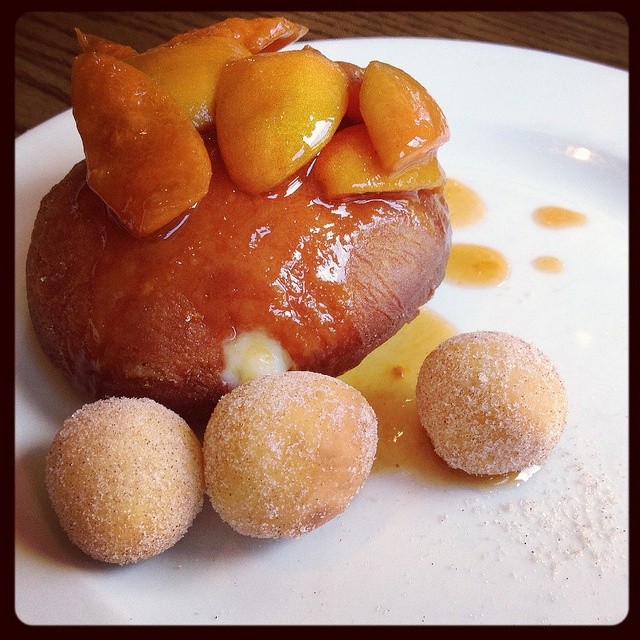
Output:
13 36 628 629
